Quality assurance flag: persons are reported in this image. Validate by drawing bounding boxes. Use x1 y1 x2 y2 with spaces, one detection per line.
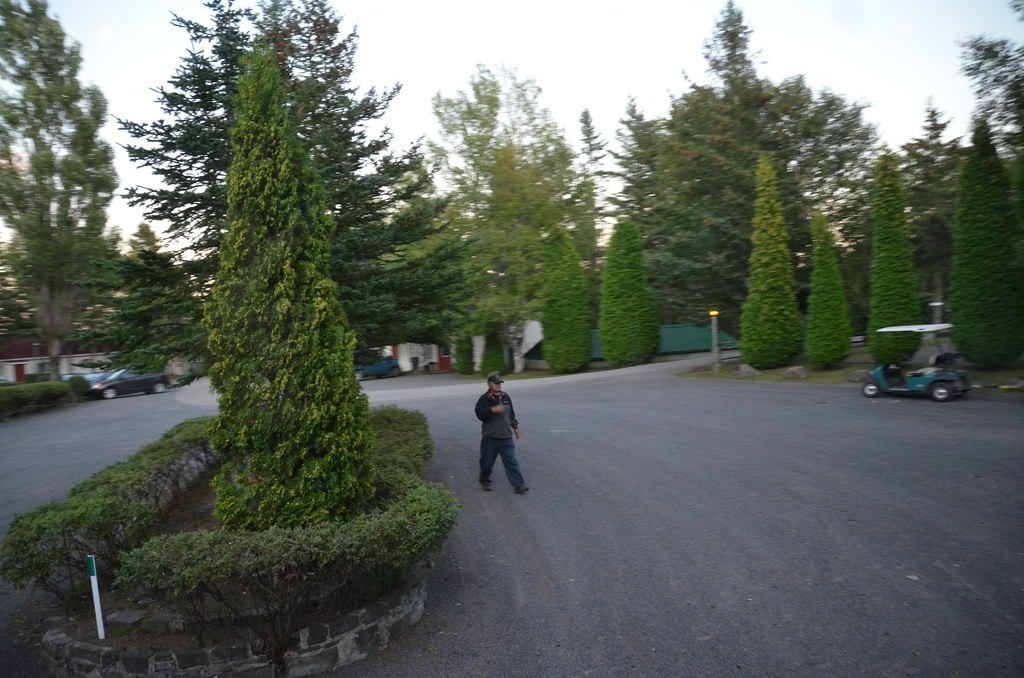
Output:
475 373 529 494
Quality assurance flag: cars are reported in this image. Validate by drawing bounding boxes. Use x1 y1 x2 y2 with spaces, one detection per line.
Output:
52 364 172 401
351 348 401 381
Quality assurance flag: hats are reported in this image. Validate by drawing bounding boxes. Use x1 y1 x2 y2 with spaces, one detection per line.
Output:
487 371 504 383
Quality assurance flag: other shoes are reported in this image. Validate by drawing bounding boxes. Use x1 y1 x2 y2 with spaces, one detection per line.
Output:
514 486 529 493
483 484 492 491
